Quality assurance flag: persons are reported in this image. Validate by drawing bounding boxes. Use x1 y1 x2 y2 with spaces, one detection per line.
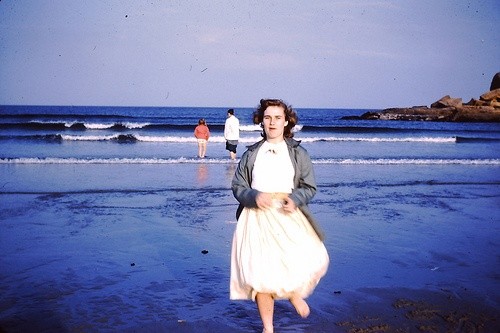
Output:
231 97 329 333
194 119 210 158
224 109 239 159
